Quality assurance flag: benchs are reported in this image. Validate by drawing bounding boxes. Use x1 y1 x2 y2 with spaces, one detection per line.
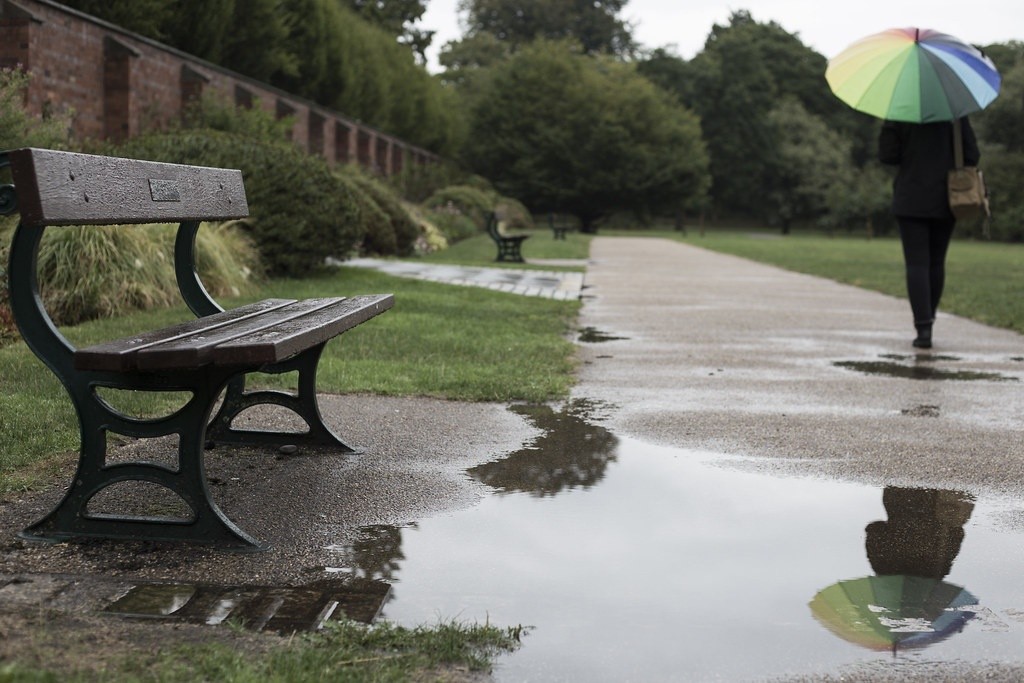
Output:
0 147 399 549
487 205 532 262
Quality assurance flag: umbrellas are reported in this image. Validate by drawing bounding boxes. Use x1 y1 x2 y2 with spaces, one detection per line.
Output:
824 27 999 123
809 574 980 658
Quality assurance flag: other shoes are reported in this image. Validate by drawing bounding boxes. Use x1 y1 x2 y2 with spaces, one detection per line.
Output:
912 337 932 349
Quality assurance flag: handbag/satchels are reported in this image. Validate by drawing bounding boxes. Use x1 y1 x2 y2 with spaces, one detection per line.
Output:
948 166 992 241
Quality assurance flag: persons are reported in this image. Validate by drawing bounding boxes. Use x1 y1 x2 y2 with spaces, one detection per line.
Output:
879 116 981 349
865 487 976 575
